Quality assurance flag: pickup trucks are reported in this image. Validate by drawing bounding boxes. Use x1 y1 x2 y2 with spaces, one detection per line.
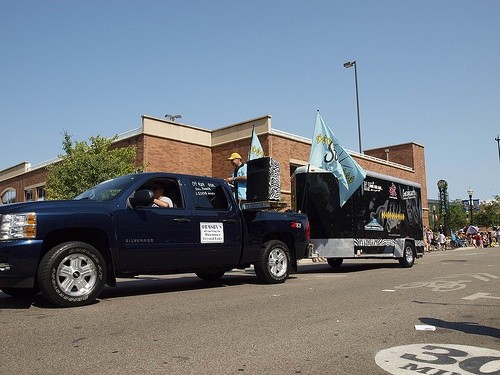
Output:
1 170 313 307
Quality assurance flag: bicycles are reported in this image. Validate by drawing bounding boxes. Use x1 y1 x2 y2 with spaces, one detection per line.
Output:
440 239 448 252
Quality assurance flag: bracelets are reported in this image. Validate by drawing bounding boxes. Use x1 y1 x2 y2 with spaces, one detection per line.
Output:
234 177 235 180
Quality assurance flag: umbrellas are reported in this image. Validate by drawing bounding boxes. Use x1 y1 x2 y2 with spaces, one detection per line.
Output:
463 225 480 234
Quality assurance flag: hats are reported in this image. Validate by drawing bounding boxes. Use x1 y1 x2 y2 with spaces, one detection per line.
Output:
228 152 242 160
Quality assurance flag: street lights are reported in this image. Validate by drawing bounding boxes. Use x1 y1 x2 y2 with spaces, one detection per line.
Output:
467 188 474 243
343 60 363 153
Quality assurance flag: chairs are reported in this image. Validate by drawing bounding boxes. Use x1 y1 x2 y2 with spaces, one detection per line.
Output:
163 184 178 209
429 243 441 251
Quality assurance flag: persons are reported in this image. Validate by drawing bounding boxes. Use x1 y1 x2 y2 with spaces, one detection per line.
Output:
423 225 500 251
227 153 247 200
151 182 173 208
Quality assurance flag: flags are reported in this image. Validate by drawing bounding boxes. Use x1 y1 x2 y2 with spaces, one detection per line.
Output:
309 111 366 208
249 128 264 161
